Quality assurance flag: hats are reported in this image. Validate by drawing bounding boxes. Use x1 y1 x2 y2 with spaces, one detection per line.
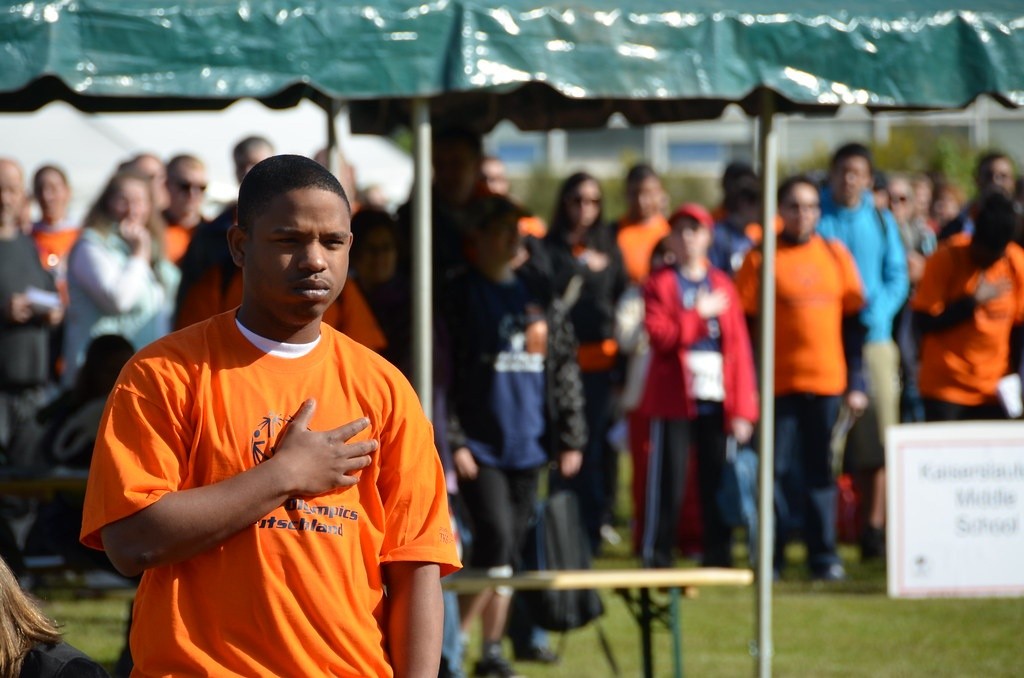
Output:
448 193 533 234
667 203 716 232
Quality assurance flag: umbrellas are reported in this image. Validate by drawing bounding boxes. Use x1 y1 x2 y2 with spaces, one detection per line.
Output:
0 0 1024 411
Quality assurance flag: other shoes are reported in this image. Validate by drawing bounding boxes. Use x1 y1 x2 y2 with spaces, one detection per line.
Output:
859 527 886 566
808 558 850 583
513 645 560 664
471 653 516 678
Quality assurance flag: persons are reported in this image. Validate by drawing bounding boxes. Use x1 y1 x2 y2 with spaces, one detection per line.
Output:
0 137 1024 678
0 553 110 677
84 152 463 678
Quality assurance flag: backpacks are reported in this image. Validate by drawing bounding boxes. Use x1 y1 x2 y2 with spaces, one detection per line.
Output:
513 488 606 633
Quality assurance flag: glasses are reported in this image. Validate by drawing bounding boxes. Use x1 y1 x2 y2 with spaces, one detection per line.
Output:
175 182 207 194
568 197 601 207
890 195 912 202
